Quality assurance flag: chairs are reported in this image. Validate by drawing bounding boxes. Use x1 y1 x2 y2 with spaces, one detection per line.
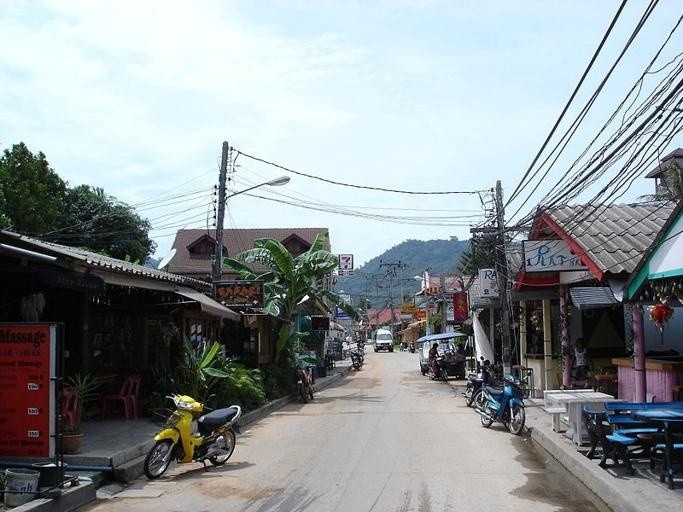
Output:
103 373 143 421
57 387 78 429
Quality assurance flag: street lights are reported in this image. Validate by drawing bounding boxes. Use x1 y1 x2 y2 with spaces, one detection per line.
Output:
415 275 441 288
223 175 290 202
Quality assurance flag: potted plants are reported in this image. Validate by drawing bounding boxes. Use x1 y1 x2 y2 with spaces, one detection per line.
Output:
58 373 103 454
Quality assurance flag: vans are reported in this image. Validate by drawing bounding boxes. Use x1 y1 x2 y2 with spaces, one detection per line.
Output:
372 329 393 352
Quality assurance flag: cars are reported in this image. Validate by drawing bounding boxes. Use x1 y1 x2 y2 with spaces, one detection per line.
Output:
422 340 455 358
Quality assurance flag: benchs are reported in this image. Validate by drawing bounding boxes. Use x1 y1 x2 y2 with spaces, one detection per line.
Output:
582 401 683 489
543 386 614 445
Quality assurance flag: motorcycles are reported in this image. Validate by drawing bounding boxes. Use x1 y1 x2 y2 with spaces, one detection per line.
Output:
462 356 531 435
297 364 317 403
410 342 415 353
425 356 448 381
350 340 364 371
143 393 242 479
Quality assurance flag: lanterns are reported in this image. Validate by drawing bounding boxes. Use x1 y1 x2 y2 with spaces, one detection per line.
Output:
650 305 674 328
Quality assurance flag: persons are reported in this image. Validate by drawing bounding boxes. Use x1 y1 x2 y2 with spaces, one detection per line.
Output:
571 337 595 381
428 342 440 375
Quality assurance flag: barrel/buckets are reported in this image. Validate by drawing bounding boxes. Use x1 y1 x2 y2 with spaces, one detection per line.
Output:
5 465 39 506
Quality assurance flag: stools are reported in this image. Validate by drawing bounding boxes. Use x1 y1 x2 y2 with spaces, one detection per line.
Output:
521 368 534 398
513 365 524 382
